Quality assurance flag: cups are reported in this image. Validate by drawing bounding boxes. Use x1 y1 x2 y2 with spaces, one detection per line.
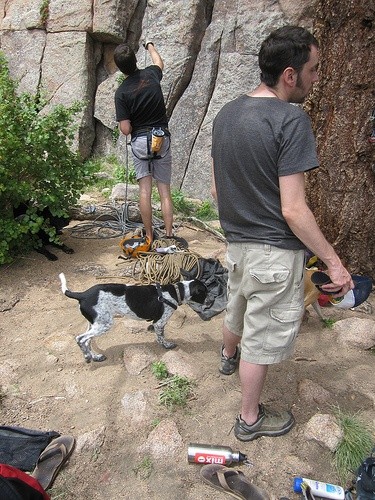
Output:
151 129 164 151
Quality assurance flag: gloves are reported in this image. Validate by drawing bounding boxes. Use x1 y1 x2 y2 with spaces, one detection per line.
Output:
142 35 154 50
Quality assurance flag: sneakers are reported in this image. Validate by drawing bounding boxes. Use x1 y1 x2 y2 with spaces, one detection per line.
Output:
234 403 295 442
219 344 240 374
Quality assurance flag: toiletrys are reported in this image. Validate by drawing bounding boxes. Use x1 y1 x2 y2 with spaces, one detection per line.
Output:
293 478 345 500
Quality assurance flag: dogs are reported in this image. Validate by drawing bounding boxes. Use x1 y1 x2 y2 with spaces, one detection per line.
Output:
59 268 209 363
302 270 333 323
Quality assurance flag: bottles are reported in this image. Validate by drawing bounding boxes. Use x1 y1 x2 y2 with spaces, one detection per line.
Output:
186 442 247 466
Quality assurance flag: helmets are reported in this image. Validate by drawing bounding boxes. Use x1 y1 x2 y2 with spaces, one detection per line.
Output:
118 235 151 260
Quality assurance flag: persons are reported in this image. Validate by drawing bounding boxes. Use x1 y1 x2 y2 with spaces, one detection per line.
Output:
114 36 174 242
211 25 355 442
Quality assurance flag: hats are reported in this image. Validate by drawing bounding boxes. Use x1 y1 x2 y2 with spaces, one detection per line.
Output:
310 271 373 309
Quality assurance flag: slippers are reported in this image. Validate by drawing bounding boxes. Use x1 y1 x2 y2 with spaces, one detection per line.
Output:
200 464 270 500
31 435 76 491
